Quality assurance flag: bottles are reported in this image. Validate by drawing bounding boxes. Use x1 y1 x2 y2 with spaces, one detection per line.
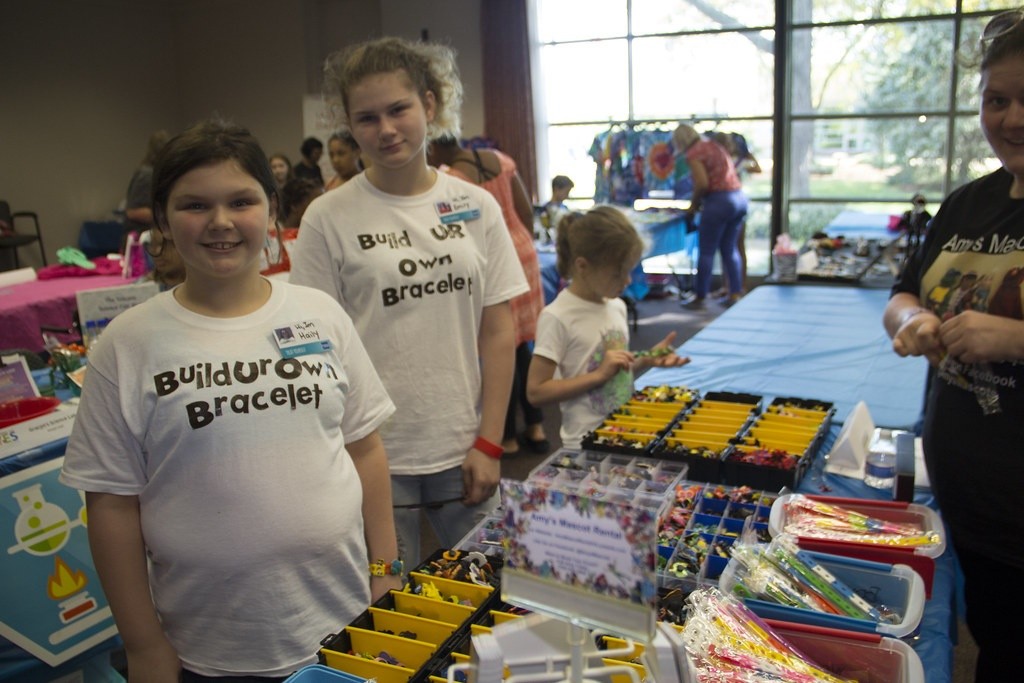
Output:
84 319 107 354
864 429 898 489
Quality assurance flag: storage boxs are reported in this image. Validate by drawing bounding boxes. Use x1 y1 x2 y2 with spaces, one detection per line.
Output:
757 619 924 683
717 544 926 647
768 493 947 559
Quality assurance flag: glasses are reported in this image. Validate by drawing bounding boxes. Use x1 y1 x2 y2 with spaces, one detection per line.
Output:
980 9 1024 57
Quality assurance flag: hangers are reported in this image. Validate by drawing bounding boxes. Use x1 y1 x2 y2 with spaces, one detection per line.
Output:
608 115 730 133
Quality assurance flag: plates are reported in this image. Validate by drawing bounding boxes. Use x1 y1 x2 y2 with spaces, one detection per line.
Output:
66 365 86 388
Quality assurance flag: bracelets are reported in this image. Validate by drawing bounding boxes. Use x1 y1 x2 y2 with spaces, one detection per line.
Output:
369 557 404 578
473 435 505 460
904 310 930 321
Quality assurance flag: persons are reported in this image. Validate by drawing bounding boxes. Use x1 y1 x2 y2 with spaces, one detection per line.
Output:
884 10 1024 682
525 206 692 448
540 175 574 215
425 132 551 457
58 119 403 683
267 129 366 241
289 35 532 575
124 131 176 254
674 124 761 310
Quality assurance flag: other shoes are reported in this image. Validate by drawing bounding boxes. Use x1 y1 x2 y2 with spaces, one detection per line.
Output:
517 430 550 455
680 300 707 312
502 449 520 459
719 299 739 306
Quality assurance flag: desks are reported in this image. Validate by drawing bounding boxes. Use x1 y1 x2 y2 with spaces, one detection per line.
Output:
0 206 959 683
